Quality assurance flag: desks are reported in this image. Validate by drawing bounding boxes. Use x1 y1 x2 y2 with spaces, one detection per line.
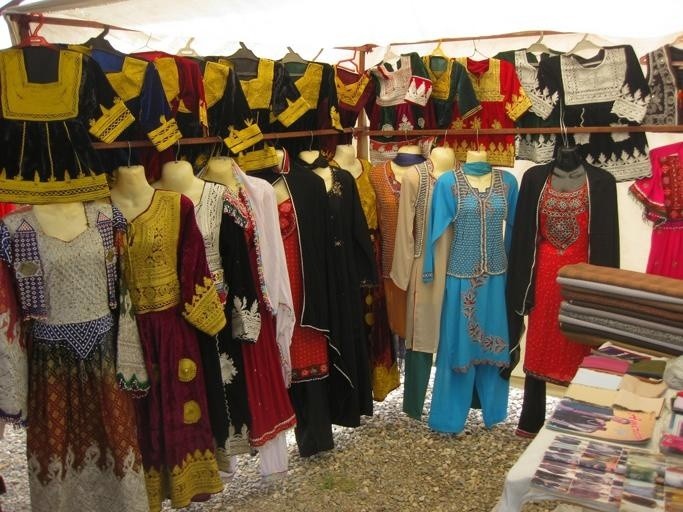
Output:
485 353 683 512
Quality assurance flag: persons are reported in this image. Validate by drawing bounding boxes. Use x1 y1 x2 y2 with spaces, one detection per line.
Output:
0 145 665 512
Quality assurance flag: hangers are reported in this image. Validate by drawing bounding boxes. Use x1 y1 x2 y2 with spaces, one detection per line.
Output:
4 9 683 86
